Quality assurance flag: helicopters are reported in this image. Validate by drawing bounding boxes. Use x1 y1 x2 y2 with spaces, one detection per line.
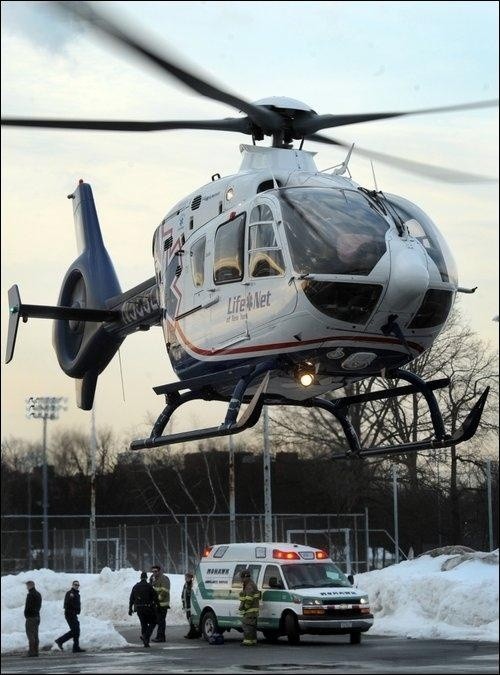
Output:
0 0 500 462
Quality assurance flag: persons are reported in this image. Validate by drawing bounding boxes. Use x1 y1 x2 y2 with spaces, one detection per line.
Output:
129 572 160 648
149 565 172 642
55 581 87 652
182 572 203 639
239 570 261 647
24 581 42 657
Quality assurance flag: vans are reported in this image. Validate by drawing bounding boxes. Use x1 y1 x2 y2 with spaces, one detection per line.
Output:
190 542 375 646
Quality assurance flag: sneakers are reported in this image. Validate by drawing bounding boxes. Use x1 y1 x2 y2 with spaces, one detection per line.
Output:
72 648 86 653
140 636 165 647
26 654 39 657
240 642 257 646
55 639 63 649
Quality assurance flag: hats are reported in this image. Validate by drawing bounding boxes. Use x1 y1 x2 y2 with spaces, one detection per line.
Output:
241 571 250 578
141 572 147 578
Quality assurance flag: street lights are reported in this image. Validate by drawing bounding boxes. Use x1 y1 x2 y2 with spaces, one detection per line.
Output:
29 397 69 567
